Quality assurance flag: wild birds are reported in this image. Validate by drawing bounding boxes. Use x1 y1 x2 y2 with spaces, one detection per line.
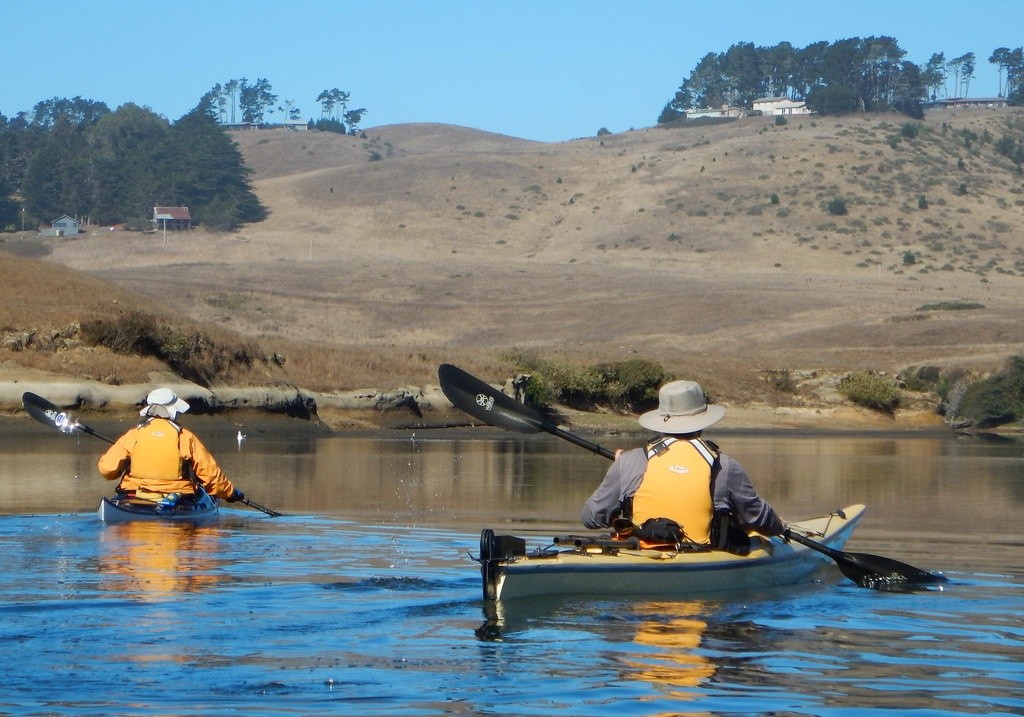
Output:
237 431 247 440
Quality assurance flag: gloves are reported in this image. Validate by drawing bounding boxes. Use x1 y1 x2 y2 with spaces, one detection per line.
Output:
225 489 244 503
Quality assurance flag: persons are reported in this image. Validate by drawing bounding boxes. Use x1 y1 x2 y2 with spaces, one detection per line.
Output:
582 380 787 557
98 387 244 512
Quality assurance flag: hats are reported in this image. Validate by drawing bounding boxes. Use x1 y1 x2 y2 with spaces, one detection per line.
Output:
638 380 725 433
139 388 190 420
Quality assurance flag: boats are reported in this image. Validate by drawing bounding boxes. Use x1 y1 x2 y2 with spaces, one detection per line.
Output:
95 483 221 523
463 503 869 624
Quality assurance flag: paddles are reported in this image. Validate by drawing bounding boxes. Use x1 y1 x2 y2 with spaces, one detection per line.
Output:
432 362 949 596
21 390 285 517
